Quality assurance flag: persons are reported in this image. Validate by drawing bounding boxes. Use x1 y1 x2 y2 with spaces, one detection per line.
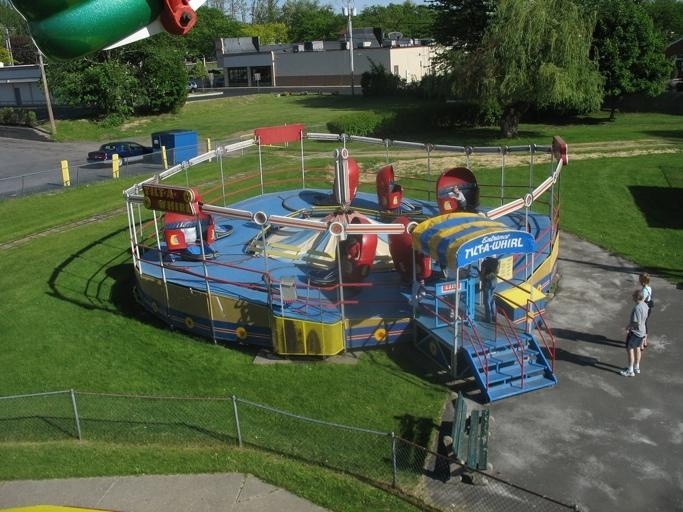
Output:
619 290 648 377
638 272 652 350
342 237 361 275
451 185 467 212
479 256 499 324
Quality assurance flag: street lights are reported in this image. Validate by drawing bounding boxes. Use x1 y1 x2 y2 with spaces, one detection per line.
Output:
342 0 357 96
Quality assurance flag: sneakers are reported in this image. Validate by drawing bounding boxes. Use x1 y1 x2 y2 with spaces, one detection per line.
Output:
619 368 640 376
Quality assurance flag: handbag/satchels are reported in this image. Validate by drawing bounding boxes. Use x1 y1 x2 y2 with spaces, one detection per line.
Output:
644 300 654 307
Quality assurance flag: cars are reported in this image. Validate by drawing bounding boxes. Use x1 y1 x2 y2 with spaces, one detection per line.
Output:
87 141 152 167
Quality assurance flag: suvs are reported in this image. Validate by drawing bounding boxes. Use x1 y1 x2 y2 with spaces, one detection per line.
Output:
186 81 198 94
